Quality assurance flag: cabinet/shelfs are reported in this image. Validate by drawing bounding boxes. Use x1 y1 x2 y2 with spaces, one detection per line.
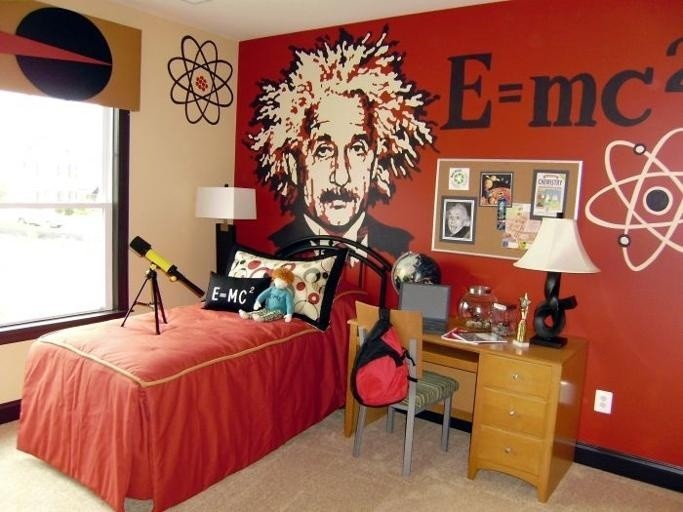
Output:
344 315 589 503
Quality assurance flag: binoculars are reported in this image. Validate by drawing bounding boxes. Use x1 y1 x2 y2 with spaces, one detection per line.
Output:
117 236 205 336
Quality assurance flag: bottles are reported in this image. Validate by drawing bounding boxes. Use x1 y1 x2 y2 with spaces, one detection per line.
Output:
458 285 498 332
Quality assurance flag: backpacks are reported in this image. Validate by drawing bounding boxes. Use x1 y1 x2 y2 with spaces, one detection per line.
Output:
348 304 411 408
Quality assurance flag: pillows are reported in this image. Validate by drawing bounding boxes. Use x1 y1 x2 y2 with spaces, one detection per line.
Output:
223 242 349 335
200 271 273 313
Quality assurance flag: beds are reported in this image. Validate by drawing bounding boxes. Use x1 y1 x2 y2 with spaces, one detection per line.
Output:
15 234 390 512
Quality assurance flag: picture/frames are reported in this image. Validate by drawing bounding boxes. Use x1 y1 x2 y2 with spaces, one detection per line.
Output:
439 196 477 244
479 172 513 208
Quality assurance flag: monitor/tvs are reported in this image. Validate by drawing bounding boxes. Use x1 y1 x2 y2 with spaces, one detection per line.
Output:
398 281 452 335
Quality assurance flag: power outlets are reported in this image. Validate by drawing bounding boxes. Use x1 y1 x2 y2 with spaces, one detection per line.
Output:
594 389 613 415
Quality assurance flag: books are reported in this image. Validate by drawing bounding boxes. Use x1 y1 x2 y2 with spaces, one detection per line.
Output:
451 331 508 345
441 325 479 347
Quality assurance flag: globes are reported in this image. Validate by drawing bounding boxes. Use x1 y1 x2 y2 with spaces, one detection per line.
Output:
391 250 442 296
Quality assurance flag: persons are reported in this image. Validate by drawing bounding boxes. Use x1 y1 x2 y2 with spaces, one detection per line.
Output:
250 29 439 257
445 203 470 239
238 265 294 324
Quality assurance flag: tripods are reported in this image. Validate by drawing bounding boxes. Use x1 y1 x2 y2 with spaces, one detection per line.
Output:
121 267 168 335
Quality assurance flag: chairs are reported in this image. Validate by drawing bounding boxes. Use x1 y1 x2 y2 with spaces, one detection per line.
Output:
352 299 460 473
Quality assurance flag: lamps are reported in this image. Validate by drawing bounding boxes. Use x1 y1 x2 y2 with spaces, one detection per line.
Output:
513 219 600 349
195 185 257 275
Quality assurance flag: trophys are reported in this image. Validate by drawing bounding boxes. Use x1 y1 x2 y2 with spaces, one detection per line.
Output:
513 292 530 347
